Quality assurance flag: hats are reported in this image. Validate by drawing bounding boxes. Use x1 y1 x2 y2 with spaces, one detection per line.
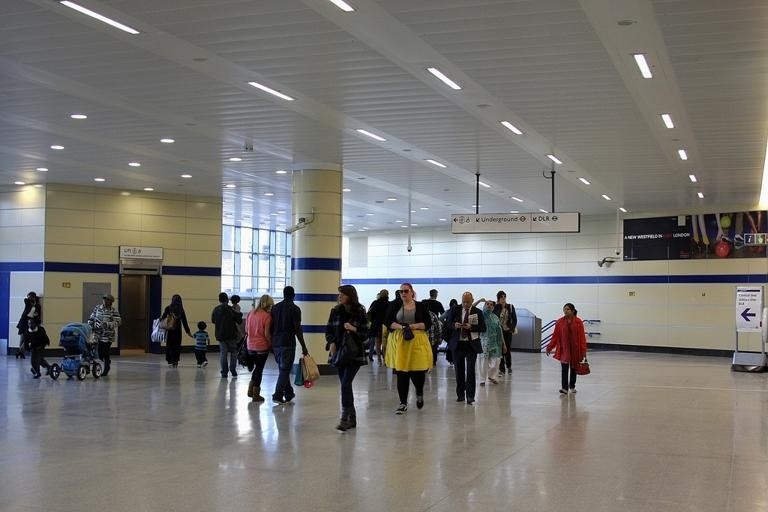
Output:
102 294 115 302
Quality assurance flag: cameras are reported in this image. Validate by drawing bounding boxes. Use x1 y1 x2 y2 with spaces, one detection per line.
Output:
399 322 408 330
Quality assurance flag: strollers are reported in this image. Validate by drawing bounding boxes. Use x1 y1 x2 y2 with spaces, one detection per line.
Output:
49 323 103 382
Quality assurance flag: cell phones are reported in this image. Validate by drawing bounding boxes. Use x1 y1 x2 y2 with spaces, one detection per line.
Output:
458 323 465 327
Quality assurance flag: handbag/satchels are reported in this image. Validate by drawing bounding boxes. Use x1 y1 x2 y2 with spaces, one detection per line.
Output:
159 311 177 329
299 353 320 380
237 350 256 371
577 355 590 375
496 324 503 347
512 326 519 336
151 319 167 342
446 331 465 364
328 344 344 369
427 310 446 347
402 325 414 341
294 363 304 386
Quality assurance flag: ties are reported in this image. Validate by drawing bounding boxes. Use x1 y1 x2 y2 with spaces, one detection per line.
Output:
462 308 470 338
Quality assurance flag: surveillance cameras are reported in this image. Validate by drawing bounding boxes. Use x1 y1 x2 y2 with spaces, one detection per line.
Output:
285 223 305 234
407 246 413 252
299 216 309 223
615 249 621 255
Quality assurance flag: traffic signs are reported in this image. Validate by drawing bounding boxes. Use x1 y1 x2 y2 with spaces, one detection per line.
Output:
737 287 760 330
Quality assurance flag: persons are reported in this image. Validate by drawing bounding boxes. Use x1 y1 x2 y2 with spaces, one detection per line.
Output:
23 318 56 380
368 282 519 413
544 303 588 396
188 321 211 369
85 294 123 376
15 292 42 359
211 286 308 404
157 294 193 370
329 428 362 511
324 284 368 432
385 394 589 511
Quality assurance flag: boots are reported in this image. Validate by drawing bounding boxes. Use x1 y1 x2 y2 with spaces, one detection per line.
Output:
248 381 295 405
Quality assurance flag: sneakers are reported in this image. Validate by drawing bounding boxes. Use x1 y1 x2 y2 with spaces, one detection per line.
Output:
347 419 356 429
202 361 208 367
571 388 576 393
416 395 424 410
33 373 42 378
368 354 387 367
480 366 513 386
336 419 347 430
559 389 568 394
197 366 201 368
395 402 407 414
